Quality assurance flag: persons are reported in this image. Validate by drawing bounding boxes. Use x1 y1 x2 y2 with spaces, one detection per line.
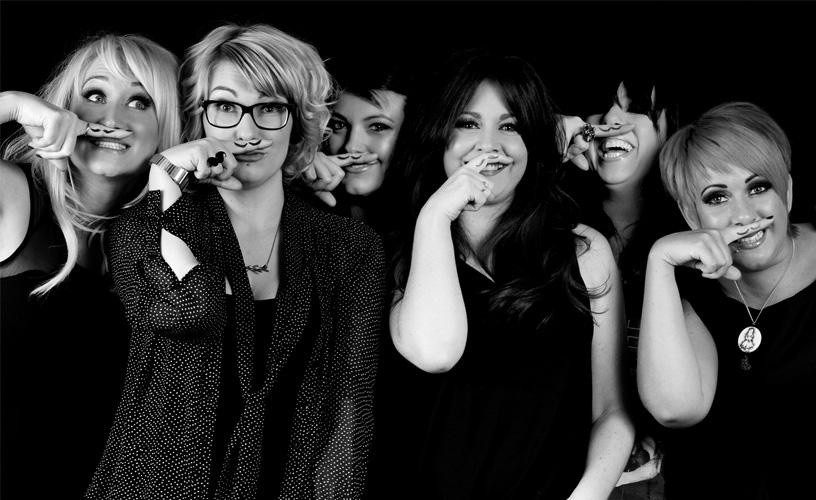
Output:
299 49 433 499
548 56 698 499
80 22 386 500
636 102 814 499
0 32 181 499
384 49 633 499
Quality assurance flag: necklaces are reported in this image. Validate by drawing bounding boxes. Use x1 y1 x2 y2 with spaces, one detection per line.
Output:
732 234 795 371
247 220 281 275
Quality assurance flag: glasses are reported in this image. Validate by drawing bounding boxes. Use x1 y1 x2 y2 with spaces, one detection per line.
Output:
196 99 294 131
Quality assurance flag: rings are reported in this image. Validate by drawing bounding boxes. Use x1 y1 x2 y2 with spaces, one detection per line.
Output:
84 122 91 137
206 152 226 169
578 124 597 143
